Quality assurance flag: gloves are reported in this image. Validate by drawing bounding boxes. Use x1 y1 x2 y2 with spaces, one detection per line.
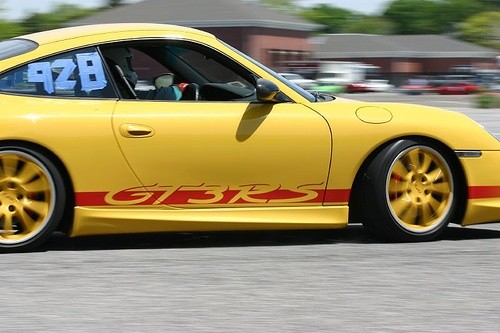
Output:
176 83 189 92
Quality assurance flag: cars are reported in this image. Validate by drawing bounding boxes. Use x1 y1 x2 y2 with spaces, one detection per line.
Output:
0 22 500 254
275 65 500 109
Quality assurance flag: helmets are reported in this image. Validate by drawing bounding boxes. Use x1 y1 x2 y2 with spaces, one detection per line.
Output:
101 43 137 89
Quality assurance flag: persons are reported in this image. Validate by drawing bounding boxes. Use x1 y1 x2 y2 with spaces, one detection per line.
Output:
108 46 188 101
